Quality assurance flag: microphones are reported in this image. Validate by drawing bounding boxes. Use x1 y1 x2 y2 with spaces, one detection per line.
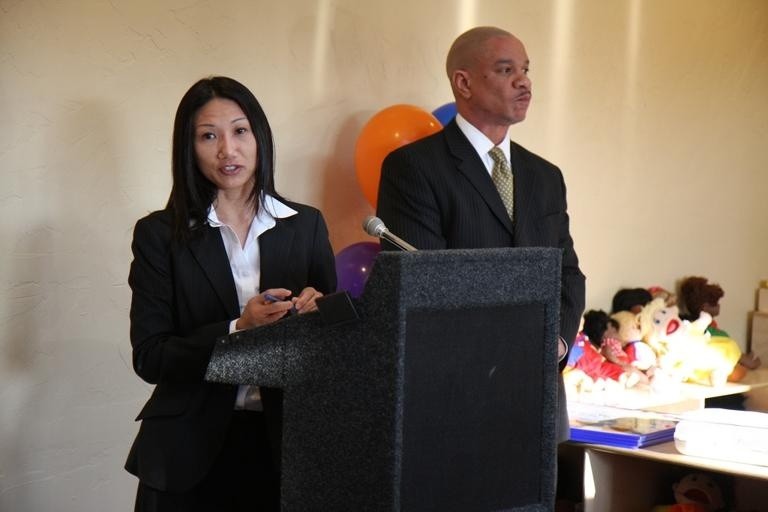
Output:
362 215 418 252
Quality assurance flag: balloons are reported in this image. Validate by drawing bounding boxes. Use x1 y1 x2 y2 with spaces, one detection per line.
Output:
334 101 458 298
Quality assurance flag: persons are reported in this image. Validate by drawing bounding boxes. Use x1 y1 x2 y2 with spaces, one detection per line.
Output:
375 27 586 469
652 472 727 512
562 277 762 398
125 76 338 512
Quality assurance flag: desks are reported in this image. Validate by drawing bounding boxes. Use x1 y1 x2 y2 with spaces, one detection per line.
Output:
563 366 768 512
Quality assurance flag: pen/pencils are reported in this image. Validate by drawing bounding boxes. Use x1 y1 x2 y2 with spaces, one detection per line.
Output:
265 295 282 303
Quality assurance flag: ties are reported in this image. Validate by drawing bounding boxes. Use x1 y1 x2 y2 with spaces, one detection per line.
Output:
488 144 515 222
